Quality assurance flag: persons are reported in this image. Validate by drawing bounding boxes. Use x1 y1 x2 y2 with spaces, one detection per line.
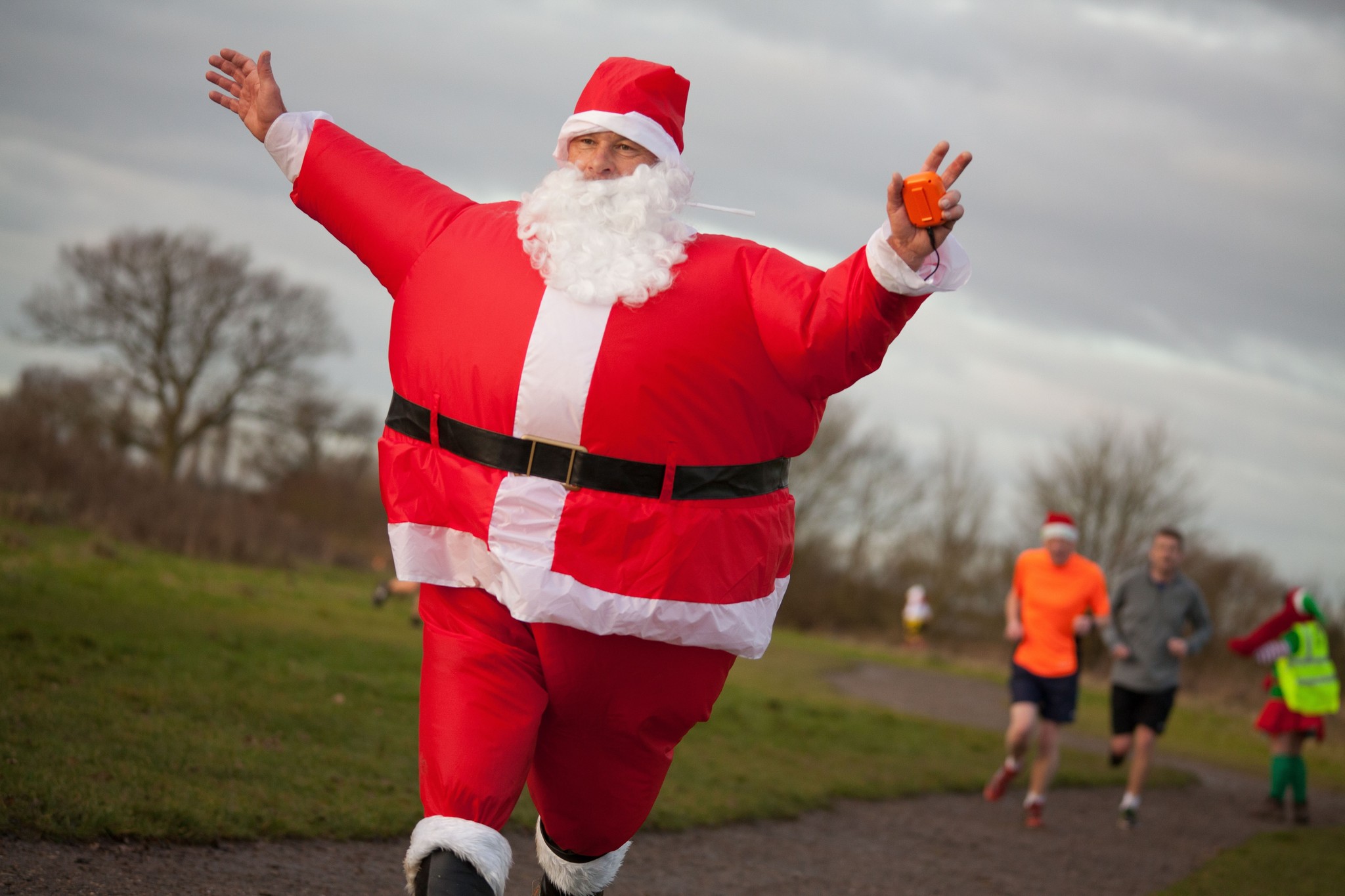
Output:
981 513 1111 828
1095 526 1213 828
205 47 973 895
1251 588 1327 825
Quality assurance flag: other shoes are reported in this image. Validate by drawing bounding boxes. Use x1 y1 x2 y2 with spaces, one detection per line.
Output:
1250 798 1287 820
1294 799 1312 822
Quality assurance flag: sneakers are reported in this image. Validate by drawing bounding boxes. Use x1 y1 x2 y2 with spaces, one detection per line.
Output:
1117 805 1139 833
984 757 1025 799
1025 799 1049 827
1110 750 1128 766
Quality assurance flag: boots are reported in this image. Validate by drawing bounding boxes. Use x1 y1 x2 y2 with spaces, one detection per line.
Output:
534 815 631 896
404 811 514 896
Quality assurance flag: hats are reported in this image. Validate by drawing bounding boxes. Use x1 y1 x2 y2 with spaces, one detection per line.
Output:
554 56 691 164
1041 510 1076 542
1286 586 1323 623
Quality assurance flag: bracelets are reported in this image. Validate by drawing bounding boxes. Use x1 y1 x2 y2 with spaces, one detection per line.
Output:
1089 616 1096 633
